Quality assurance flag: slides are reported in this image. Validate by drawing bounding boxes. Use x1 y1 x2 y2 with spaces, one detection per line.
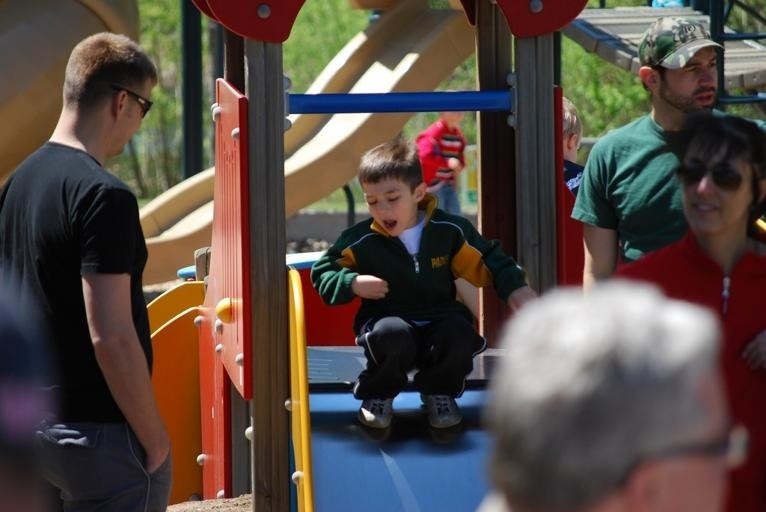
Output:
289 270 497 512
140 0 477 285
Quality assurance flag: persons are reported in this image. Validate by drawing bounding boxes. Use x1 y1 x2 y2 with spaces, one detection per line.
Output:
0 32 172 512
477 276 751 512
310 141 538 429
571 15 766 289
605 116 766 512
416 111 466 215
562 96 585 197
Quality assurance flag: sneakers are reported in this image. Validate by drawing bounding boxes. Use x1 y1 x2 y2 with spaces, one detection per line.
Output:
358 398 393 429
424 394 463 429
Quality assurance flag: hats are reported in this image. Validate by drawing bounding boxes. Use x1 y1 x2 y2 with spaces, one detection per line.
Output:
639 17 725 69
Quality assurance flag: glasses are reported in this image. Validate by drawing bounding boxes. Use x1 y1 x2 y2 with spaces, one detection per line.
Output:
109 83 153 118
684 164 743 191
616 426 748 488
